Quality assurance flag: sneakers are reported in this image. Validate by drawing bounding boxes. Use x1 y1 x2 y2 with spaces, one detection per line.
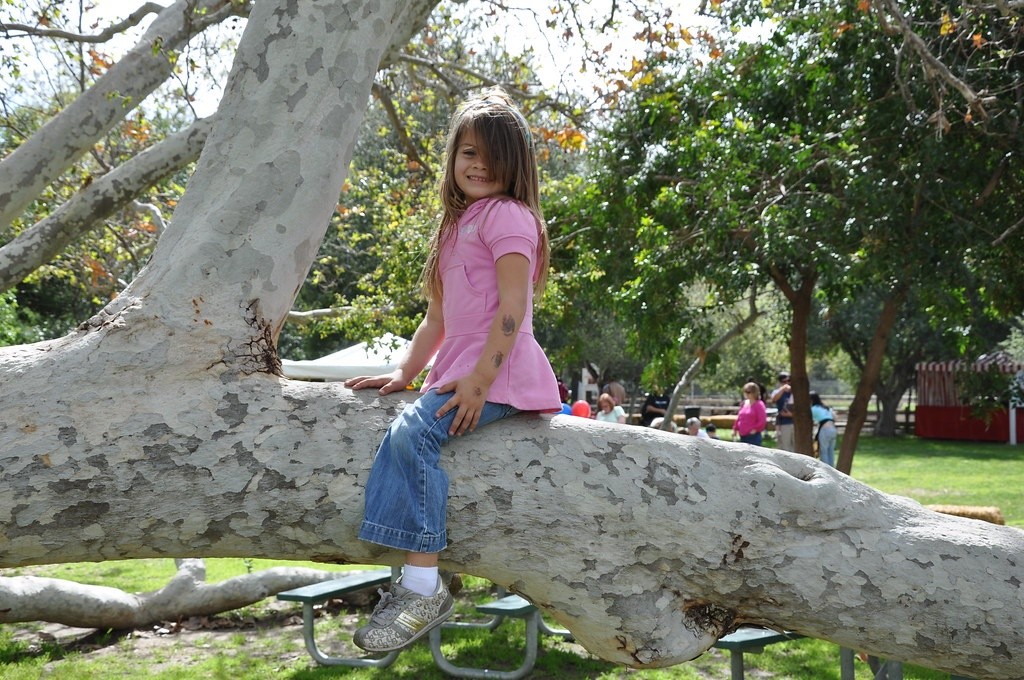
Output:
354 573 463 651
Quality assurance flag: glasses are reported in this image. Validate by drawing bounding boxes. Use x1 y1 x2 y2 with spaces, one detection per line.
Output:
745 391 751 394
782 380 791 383
600 405 610 409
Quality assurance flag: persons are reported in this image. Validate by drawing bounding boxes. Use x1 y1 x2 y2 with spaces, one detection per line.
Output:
731 382 766 445
641 383 719 442
344 88 563 652
549 370 626 424
807 390 837 468
771 372 794 452
747 376 767 405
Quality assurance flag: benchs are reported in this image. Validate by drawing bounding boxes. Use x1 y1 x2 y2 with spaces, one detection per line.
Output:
271 566 905 680
